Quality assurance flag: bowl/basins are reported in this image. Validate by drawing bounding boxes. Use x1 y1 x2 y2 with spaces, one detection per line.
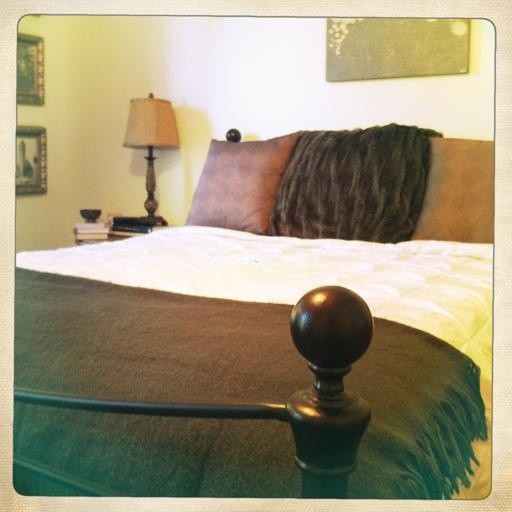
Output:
79 209 102 223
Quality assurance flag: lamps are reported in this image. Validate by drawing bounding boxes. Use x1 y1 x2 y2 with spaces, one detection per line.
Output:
124 92 180 228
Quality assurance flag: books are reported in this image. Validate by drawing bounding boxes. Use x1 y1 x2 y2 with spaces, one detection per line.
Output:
109 217 164 237
76 219 112 239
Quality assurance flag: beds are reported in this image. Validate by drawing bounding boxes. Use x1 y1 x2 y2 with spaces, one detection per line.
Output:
15 130 498 501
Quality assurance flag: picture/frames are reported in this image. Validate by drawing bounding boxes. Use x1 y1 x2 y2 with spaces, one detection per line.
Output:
17 32 46 107
16 125 48 196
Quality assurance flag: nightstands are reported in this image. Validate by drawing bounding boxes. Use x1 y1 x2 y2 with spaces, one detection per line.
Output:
108 230 144 243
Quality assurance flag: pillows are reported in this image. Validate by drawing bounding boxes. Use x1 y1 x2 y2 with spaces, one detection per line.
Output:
275 120 445 246
186 133 300 235
413 137 493 243
